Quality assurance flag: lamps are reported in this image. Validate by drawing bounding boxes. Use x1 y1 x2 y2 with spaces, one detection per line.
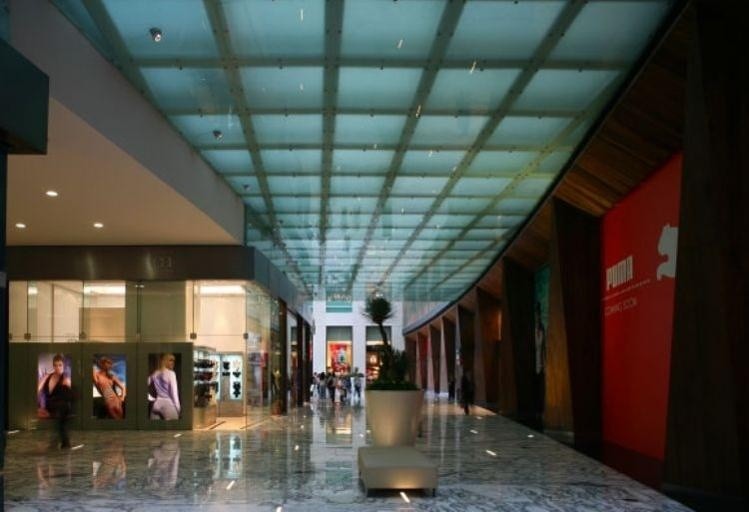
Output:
149 28 163 43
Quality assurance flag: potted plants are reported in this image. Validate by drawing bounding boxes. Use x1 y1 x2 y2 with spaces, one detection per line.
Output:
362 296 427 445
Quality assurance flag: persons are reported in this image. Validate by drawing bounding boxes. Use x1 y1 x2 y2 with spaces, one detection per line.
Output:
147 354 182 421
312 368 364 404
92 356 125 421
459 365 478 417
37 355 72 419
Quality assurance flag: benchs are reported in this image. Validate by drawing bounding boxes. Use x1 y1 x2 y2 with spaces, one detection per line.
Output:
357 445 439 498
